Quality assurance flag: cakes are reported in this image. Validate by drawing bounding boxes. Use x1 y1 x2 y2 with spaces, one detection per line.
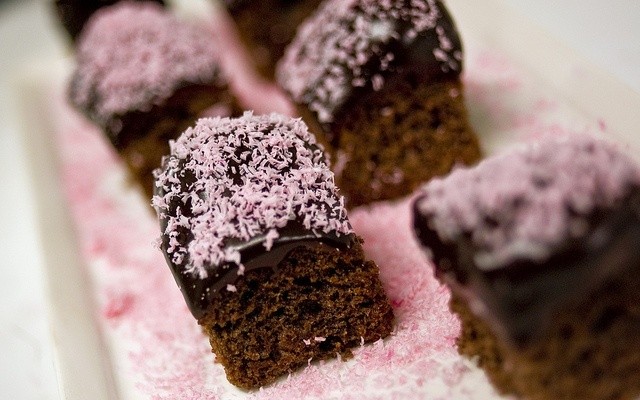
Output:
410 131 639 400
153 112 395 392
274 1 486 208
64 0 249 219
